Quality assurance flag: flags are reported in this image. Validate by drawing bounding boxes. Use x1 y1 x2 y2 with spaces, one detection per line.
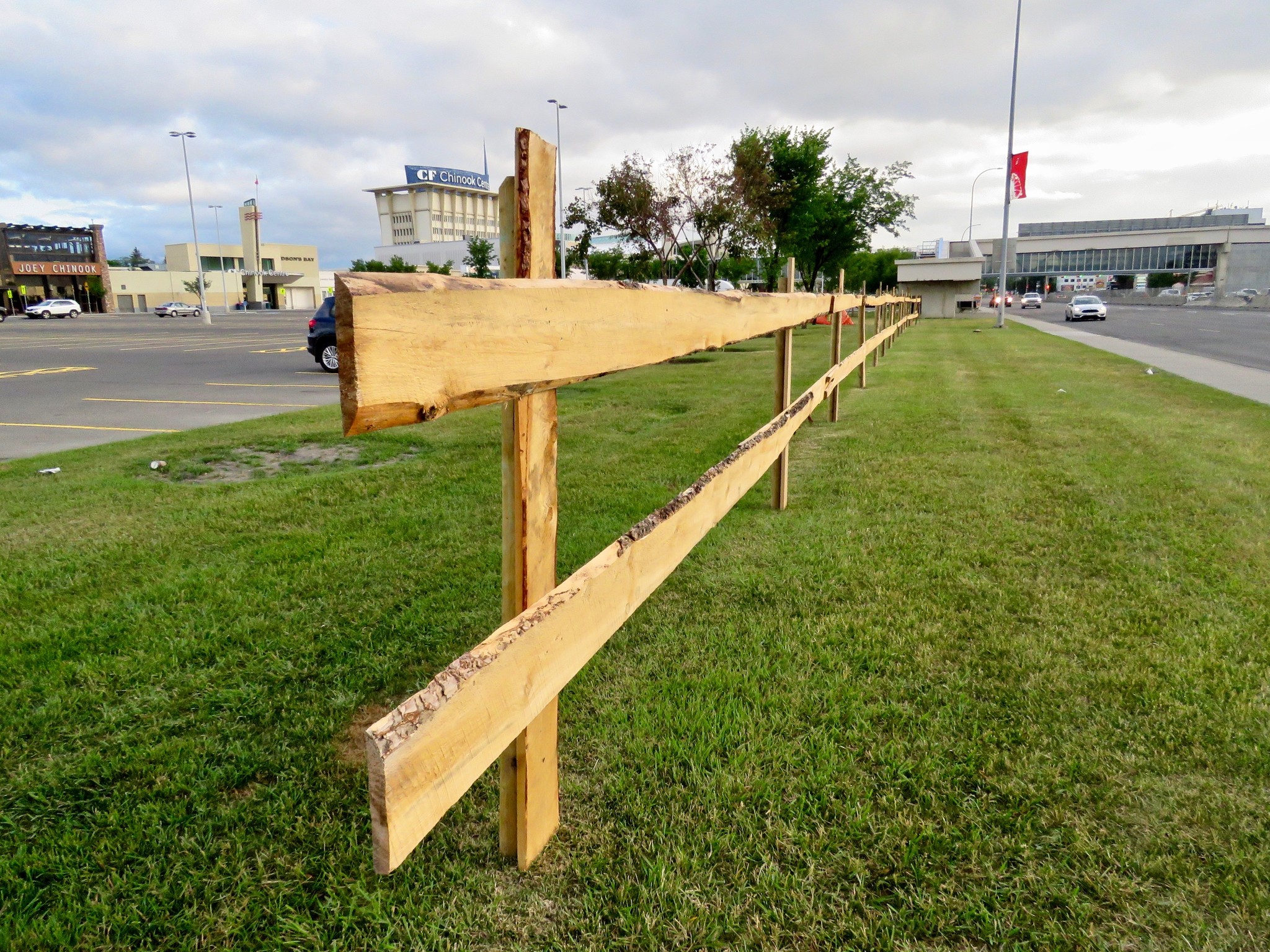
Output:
255 178 259 185
1008 151 1028 200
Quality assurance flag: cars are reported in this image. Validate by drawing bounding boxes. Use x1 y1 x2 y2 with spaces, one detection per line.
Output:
1021 293 1042 309
1184 292 1213 302
1223 288 1270 304
0 306 9 322
989 293 1011 308
1157 289 1181 297
154 302 202 318
1065 295 1107 322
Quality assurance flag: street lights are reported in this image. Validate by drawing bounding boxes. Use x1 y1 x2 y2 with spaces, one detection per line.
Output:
994 277 999 308
169 131 211 325
547 99 568 280
208 205 229 312
968 168 1003 241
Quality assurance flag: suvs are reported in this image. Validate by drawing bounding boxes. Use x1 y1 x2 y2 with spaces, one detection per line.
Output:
307 296 339 373
25 299 81 319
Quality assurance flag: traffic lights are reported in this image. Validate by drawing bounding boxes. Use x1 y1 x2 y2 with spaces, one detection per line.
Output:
1046 285 1049 290
